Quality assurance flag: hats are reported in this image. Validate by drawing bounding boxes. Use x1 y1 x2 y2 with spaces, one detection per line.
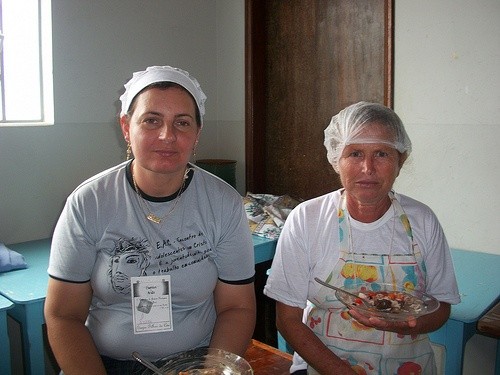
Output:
119 64 208 129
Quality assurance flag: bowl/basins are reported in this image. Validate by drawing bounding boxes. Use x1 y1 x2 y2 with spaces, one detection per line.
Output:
142 348 254 375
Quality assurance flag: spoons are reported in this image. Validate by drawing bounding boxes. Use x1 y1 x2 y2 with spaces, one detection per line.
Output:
314 277 391 312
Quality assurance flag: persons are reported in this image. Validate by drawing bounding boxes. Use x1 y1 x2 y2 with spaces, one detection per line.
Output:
261 100 464 375
40 65 258 375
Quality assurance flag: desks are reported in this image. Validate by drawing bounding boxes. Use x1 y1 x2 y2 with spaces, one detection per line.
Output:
266 247 499 375
0 238 276 375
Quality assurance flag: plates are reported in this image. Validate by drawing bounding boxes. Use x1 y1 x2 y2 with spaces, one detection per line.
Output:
334 281 440 321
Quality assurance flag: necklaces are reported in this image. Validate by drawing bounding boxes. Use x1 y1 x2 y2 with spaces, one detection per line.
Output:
129 160 189 264
